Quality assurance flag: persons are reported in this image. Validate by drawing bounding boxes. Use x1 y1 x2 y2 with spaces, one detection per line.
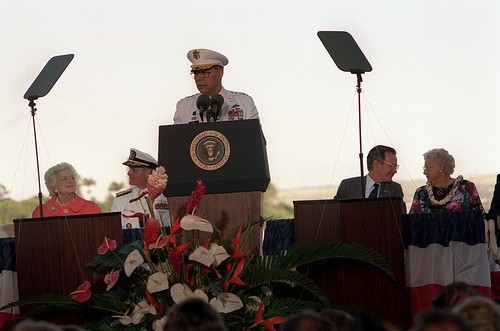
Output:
32 162 102 218
173 48 261 124
163 282 500 331
409 149 485 216
111 148 171 229
333 145 406 214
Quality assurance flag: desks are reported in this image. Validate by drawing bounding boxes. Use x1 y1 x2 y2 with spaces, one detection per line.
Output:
293 197 407 331
0 226 171 331
261 211 492 331
13 212 124 326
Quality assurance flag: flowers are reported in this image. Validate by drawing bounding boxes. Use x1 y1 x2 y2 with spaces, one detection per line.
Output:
0 166 399 331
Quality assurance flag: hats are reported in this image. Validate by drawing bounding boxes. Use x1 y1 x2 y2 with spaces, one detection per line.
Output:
122 148 158 171
187 49 229 70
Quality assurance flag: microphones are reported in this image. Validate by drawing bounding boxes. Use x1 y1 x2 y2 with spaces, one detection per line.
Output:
210 94 224 115
197 94 211 114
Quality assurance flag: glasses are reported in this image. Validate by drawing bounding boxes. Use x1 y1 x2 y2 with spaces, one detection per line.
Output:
423 166 433 171
190 69 219 78
376 159 399 170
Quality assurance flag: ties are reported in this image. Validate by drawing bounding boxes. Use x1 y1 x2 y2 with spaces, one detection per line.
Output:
367 183 379 199
